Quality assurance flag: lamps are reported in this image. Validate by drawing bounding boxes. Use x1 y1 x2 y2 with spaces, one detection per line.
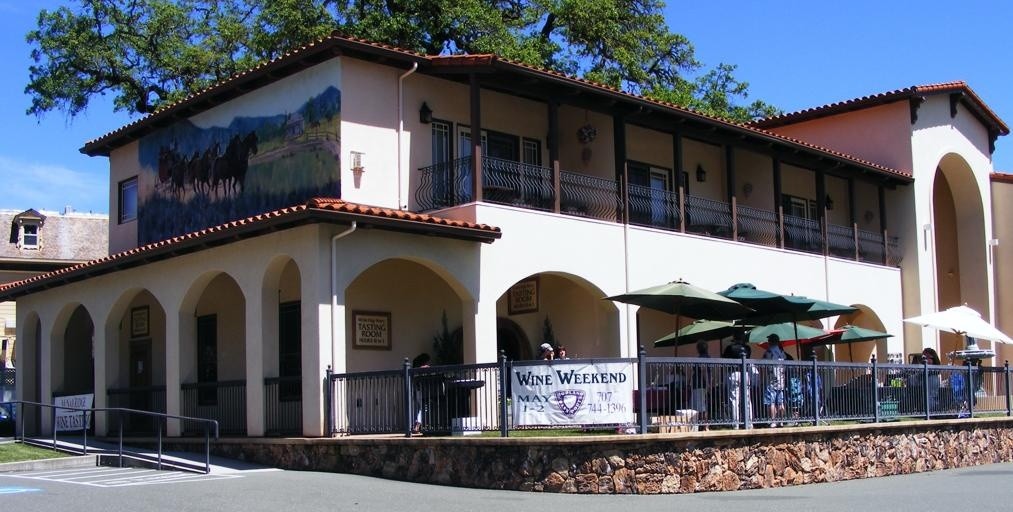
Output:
420 101 433 124
696 163 707 182
825 194 834 211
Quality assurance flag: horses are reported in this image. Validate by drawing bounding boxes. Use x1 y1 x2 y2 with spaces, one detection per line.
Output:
157 131 258 202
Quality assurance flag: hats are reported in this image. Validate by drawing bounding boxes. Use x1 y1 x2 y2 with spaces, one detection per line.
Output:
730 330 751 343
540 342 553 352
766 333 780 342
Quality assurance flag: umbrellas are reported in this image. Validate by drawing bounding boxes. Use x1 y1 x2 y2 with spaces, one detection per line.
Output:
902 298 1013 382
598 276 859 361
812 322 894 381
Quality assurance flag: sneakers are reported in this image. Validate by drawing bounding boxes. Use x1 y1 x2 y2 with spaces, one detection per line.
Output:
702 419 799 429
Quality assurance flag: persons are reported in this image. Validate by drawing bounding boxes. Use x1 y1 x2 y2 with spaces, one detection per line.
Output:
802 357 825 418
688 340 713 431
721 331 755 429
539 342 554 360
782 351 804 427
552 344 570 360
409 347 434 436
758 333 786 428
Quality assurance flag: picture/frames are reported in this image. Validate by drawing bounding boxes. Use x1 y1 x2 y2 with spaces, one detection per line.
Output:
352 310 391 350
508 277 539 316
131 304 150 338
